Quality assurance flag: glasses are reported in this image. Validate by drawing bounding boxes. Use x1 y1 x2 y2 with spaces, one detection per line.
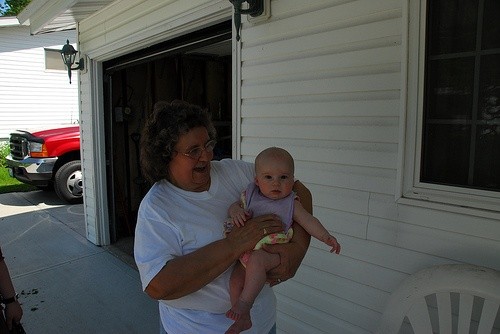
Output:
175 138 217 159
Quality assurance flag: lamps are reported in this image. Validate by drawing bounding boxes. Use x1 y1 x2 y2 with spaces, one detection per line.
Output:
58 39 84 84
229 0 264 42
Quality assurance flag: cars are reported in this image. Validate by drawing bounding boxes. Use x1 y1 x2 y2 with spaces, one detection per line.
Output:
3 126 83 204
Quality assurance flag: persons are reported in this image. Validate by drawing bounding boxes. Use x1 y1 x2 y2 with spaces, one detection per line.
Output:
134 98 313 334
0 247 27 334
224 147 341 334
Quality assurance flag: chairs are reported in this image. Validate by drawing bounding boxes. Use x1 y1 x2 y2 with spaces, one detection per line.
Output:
377 264 500 334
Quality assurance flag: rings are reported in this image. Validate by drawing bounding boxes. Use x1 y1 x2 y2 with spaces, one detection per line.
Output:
263 227 267 235
277 279 281 283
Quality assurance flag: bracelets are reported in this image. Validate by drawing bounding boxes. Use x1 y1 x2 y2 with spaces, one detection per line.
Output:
3 294 19 305
